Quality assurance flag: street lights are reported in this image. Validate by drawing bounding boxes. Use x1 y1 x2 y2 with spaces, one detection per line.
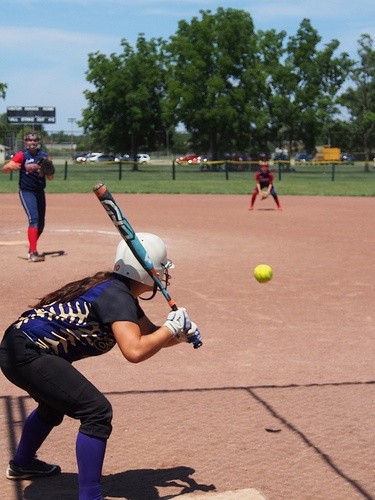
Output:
68 117 77 153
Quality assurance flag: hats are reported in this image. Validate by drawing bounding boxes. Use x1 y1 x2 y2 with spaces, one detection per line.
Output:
259 161 269 166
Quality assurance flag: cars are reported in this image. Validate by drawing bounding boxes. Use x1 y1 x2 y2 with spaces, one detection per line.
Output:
72 151 151 166
175 149 355 172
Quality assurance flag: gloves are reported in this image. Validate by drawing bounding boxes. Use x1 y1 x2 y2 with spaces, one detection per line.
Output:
180 321 201 345
165 307 191 338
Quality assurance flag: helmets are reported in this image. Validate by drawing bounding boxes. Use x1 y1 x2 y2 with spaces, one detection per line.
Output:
112 232 176 291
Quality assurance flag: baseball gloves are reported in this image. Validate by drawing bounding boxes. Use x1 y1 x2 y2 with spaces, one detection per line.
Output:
36 157 55 176
261 191 268 201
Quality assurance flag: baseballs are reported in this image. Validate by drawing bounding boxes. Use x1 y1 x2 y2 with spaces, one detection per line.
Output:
253 263 273 283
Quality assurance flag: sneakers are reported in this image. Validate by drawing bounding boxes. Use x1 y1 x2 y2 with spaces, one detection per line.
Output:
6 455 61 480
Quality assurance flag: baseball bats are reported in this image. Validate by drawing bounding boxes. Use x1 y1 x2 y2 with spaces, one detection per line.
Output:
92 182 203 349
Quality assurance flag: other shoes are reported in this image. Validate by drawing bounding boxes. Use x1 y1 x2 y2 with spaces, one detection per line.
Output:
28 249 43 261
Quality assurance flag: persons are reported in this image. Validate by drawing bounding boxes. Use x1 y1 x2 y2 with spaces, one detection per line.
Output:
249 158 282 211
0 231 202 500
3 132 54 261
